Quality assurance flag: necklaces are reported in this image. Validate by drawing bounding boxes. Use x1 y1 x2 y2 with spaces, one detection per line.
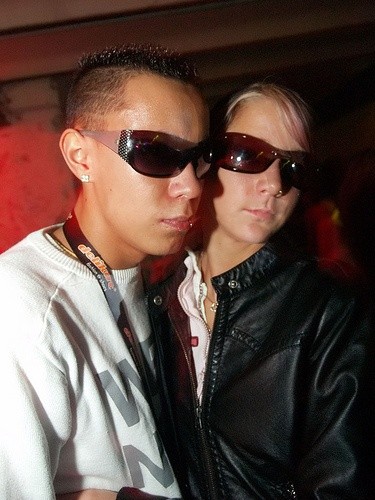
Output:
197 280 218 341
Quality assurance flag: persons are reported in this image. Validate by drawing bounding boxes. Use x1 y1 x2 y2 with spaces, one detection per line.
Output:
0 44 212 500
50 72 374 500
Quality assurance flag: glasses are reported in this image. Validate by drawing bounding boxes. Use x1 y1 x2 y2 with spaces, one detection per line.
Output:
77 124 220 184
209 130 316 194
